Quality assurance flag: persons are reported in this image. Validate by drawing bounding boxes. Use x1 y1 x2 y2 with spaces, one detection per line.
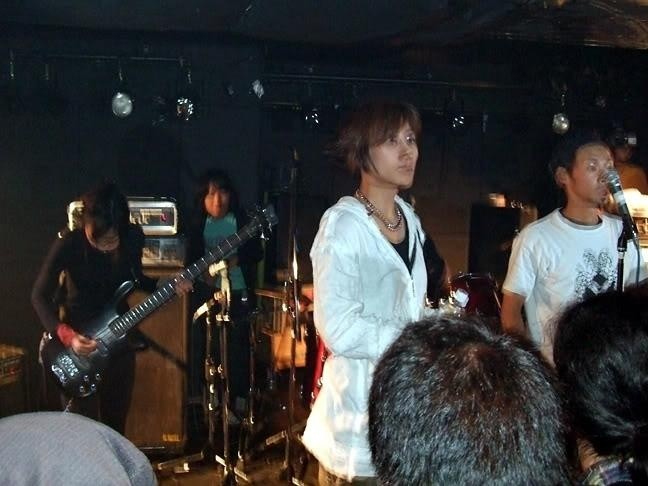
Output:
368 315 572 486
29 182 195 437
187 171 263 450
556 294 648 486
500 132 648 366
296 101 475 486
605 133 648 215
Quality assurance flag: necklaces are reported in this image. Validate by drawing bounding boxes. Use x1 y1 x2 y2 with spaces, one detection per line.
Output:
354 190 403 231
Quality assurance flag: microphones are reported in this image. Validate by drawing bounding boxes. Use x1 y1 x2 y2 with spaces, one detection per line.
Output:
604 170 639 241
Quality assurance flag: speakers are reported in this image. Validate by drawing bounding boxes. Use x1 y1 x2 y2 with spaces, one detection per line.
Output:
466 201 522 292
108 269 194 457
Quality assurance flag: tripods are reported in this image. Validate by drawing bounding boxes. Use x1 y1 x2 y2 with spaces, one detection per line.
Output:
152 304 318 486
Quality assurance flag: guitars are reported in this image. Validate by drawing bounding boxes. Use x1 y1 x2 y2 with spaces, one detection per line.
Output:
40 200 280 399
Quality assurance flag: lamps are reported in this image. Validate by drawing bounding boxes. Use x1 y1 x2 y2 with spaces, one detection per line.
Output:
250 68 570 136
0 44 201 123
